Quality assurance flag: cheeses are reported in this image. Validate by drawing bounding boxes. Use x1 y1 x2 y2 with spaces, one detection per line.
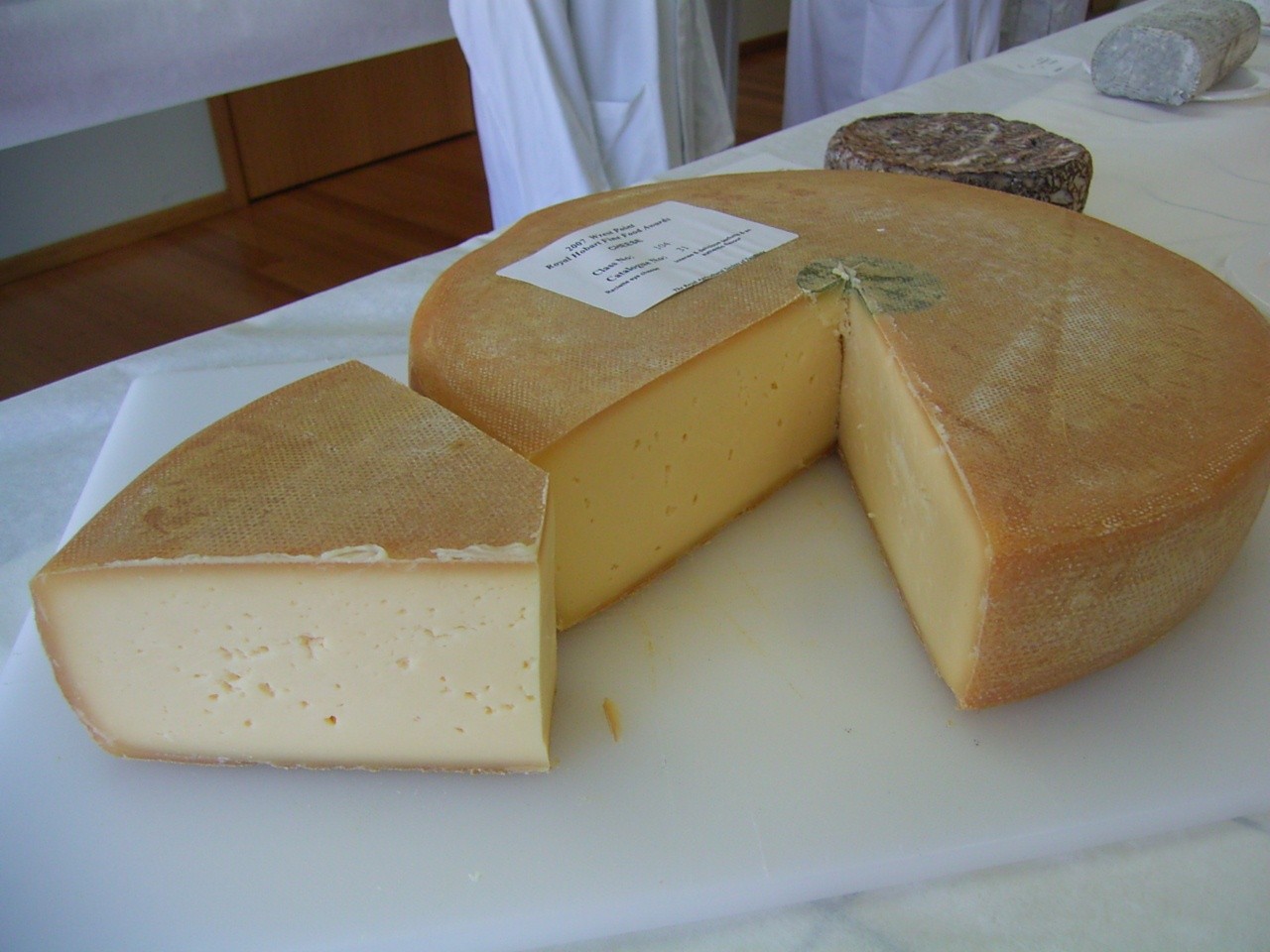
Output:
36 171 1269 779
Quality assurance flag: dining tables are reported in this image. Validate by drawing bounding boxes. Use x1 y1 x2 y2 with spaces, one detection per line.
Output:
1 1 1270 952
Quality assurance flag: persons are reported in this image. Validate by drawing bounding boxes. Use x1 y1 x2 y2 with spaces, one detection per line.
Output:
778 0 1095 131
446 0 740 232
